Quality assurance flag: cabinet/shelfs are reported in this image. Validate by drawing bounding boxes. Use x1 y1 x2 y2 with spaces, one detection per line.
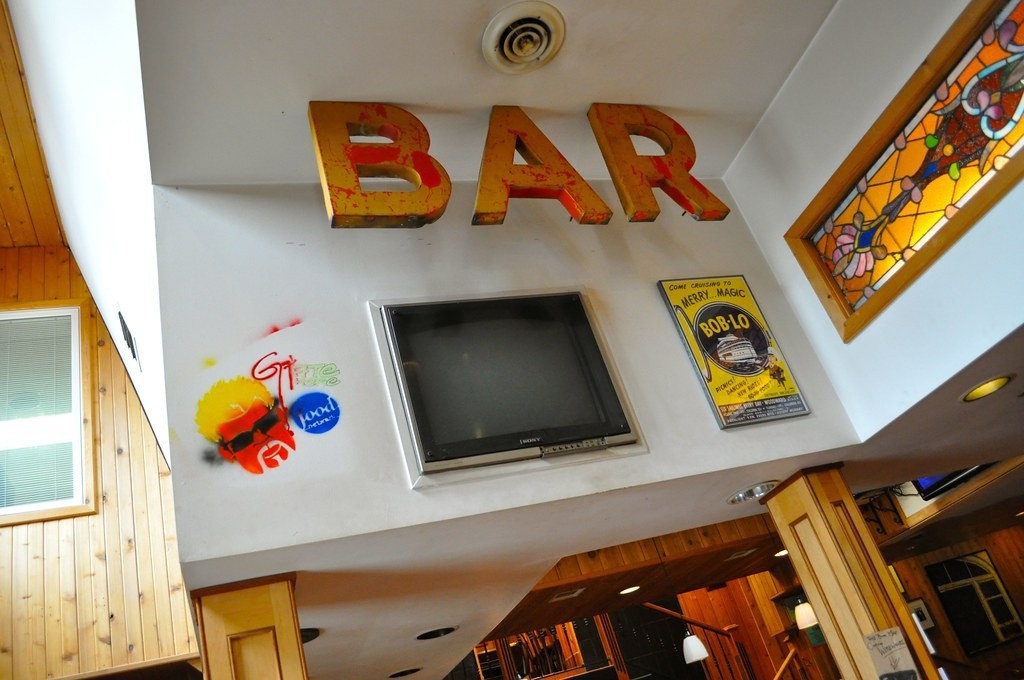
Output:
191 572 309 680
652 513 777 595
481 530 676 639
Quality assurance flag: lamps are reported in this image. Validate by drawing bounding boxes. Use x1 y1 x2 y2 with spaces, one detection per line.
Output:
683 630 708 664
794 602 818 631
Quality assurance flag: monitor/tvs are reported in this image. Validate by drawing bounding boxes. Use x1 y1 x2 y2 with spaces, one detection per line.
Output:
911 460 999 502
381 290 638 474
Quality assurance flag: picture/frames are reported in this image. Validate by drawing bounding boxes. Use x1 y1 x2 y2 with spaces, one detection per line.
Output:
494 613 630 680
906 597 937 631
471 622 586 680
